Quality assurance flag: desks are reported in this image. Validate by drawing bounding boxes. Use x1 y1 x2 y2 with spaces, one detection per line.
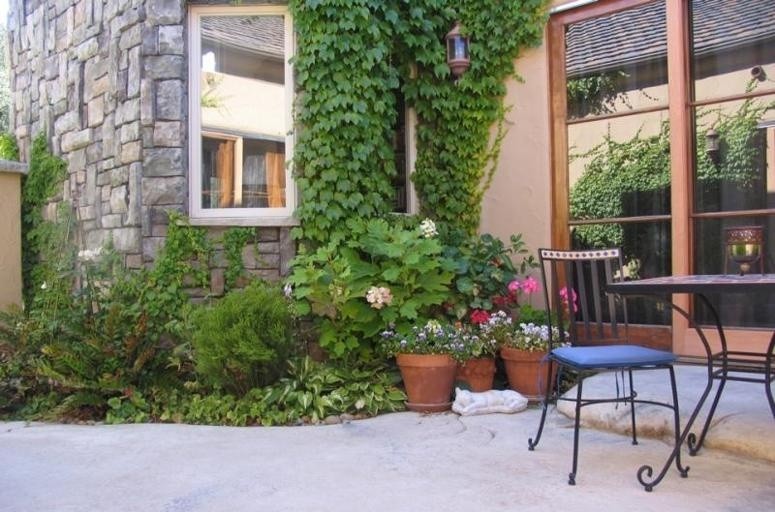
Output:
606 273 775 491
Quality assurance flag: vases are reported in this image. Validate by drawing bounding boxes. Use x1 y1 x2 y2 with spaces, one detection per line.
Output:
396 348 559 413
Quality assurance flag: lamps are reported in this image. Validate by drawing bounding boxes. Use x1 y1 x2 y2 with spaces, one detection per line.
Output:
444 20 471 76
705 129 721 156
724 225 764 276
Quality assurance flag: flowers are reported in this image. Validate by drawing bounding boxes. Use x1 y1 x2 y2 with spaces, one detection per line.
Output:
379 278 578 369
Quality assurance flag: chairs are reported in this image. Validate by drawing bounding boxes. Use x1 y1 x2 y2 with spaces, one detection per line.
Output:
528 247 691 486
